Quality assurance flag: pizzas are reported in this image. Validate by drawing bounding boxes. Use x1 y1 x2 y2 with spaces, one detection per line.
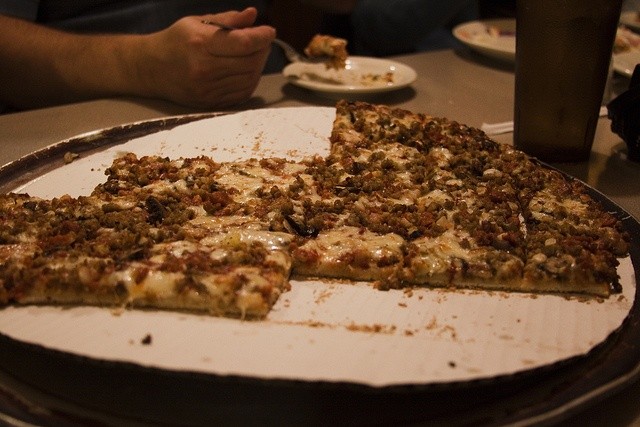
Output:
304 31 350 72
0 99 632 320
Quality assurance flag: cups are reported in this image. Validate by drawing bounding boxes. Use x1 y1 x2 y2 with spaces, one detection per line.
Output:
513 0 623 161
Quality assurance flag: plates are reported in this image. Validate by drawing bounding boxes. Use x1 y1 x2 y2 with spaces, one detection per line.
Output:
451 17 516 66
1 104 639 424
283 52 420 93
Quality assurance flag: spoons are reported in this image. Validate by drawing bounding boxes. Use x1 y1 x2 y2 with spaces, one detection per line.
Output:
199 17 329 67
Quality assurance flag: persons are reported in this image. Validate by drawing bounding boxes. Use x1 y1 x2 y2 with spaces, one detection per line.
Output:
0 7 277 110
